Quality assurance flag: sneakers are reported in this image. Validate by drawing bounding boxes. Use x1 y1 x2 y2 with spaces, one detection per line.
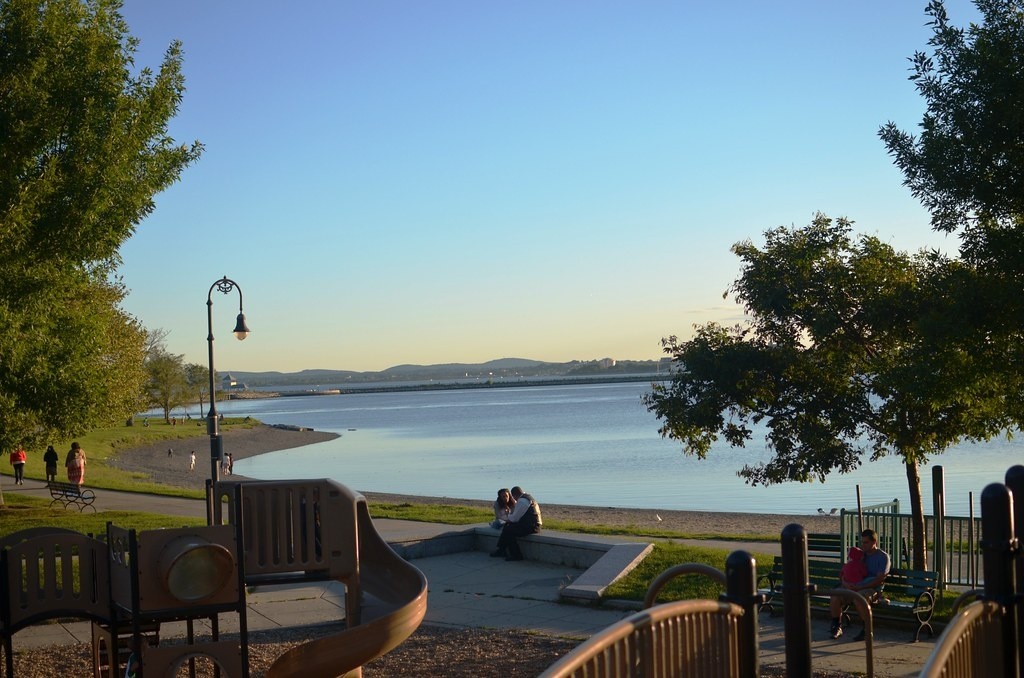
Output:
14 481 19 484
20 480 24 486
877 597 890 605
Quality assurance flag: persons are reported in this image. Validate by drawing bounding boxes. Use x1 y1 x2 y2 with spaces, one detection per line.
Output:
220 413 224 421
171 417 176 428
491 488 515 529
839 546 890 605
489 487 542 561
830 529 891 641
224 452 233 475
143 417 149 428
10 443 26 485
168 448 173 458
65 442 87 484
190 451 195 471
44 446 58 487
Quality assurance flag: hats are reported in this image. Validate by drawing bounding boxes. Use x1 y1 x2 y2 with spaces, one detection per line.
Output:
847 546 861 560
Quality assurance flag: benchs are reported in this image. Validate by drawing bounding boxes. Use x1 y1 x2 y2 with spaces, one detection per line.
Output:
755 555 940 645
807 534 908 565
47 480 97 513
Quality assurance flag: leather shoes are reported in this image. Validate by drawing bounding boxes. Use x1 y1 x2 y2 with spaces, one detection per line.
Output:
490 550 507 557
853 630 864 641
505 554 523 561
832 627 843 639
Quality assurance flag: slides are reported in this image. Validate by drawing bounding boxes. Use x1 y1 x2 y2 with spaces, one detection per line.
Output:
266 478 429 678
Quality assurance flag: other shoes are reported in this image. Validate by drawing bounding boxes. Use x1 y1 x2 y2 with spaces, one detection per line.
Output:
45 485 50 488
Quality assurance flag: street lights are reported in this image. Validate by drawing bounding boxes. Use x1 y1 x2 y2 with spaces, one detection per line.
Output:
204 274 250 495
